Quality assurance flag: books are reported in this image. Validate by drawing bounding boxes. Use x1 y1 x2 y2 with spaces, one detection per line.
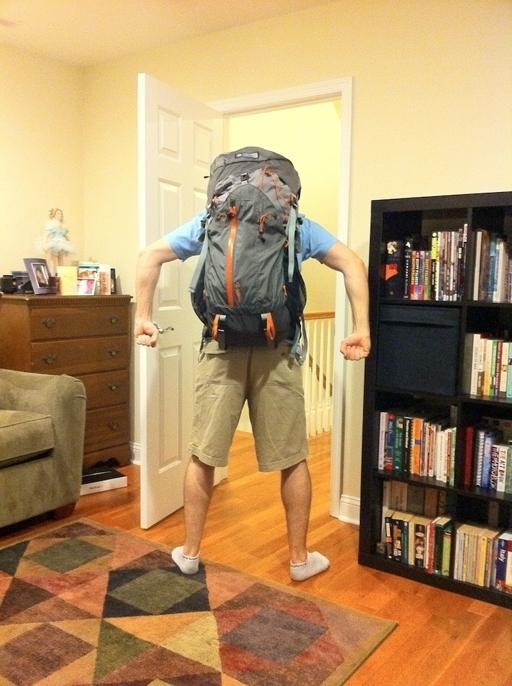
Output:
383 222 512 302
379 403 512 493
79 262 117 296
462 332 512 398
380 480 512 595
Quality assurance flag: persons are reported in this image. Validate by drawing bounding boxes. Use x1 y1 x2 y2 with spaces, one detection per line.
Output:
35 267 49 287
134 208 373 582
42 208 78 276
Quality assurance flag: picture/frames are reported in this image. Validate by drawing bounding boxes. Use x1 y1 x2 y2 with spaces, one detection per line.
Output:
23 257 58 294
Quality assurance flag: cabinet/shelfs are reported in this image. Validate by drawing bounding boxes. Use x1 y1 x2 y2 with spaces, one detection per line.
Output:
0 294 133 471
357 191 512 609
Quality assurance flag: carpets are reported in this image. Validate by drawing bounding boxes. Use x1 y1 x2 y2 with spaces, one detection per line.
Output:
1 515 399 686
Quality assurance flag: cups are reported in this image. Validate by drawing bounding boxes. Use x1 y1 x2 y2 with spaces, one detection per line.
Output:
56 266 78 296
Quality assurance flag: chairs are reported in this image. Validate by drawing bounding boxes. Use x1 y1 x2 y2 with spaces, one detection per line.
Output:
0 368 87 527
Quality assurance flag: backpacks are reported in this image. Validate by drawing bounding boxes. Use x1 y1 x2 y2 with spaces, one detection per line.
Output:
189 146 309 368
